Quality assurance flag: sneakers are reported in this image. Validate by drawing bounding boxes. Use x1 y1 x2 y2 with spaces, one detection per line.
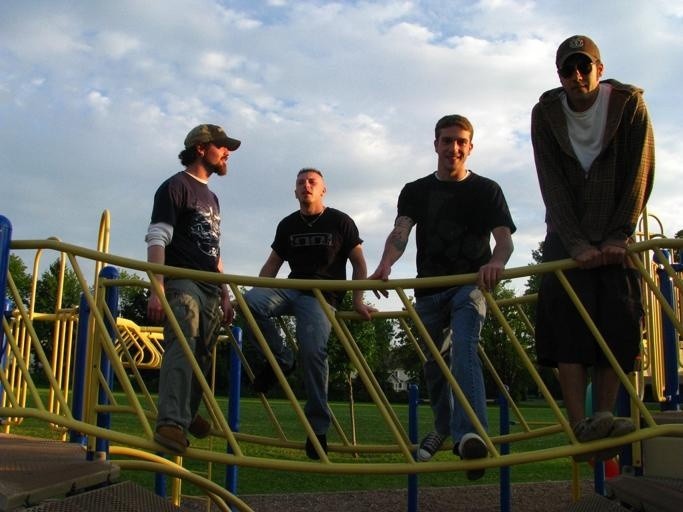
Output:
305 434 328 460
569 412 614 463
188 414 212 439
416 428 452 461
594 419 636 461
153 424 190 453
458 433 488 481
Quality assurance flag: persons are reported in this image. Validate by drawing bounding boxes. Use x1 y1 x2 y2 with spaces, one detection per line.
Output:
236 168 378 460
366 113 516 480
146 123 242 454
530 34 656 461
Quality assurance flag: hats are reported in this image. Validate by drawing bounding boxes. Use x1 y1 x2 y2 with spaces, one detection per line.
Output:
184 124 241 151
556 35 600 70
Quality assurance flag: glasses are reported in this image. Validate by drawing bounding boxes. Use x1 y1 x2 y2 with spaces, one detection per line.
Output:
557 62 594 79
